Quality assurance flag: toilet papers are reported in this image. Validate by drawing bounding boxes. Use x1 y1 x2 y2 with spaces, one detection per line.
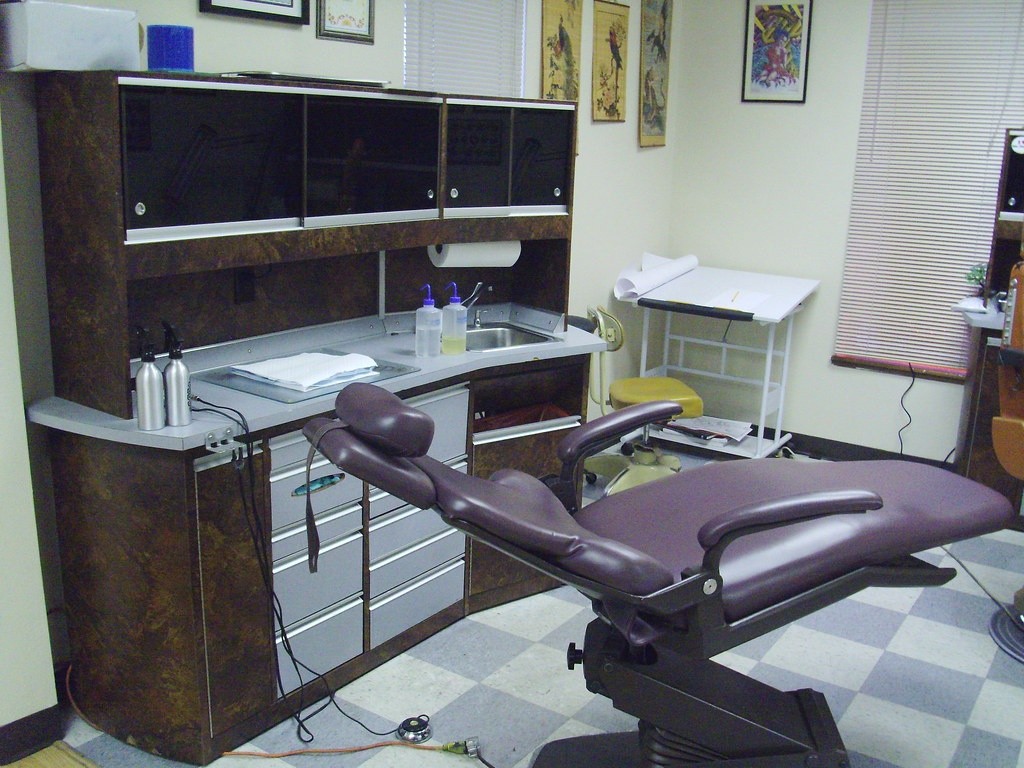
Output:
427 240 523 268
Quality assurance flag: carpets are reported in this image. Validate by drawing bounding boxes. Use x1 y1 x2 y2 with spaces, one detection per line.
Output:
60 528 1024 768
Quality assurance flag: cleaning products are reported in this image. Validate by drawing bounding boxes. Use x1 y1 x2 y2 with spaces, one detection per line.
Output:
414 281 467 359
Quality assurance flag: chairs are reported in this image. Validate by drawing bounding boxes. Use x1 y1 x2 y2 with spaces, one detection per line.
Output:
988 262 1024 664
302 384 1013 768
583 305 703 499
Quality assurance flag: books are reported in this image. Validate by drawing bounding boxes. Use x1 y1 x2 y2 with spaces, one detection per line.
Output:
649 416 754 448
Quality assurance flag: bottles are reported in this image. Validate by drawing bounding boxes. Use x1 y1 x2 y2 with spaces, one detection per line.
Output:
162 348 191 426
136 351 165 430
414 284 441 358
441 281 467 356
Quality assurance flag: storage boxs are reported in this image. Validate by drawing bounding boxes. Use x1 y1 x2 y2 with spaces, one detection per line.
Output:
0 0 144 71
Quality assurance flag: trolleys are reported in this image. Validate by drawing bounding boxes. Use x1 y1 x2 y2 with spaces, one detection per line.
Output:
619 301 803 460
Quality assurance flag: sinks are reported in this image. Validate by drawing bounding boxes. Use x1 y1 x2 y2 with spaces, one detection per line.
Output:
441 327 565 354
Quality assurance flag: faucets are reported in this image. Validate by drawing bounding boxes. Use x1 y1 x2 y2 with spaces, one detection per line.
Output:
471 309 488 329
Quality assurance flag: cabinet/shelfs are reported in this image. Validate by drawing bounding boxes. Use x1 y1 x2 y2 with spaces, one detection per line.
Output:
618 298 804 459
43 352 591 767
33 70 578 418
955 128 1024 534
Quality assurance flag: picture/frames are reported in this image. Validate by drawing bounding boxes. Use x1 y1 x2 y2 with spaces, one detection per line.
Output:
315 0 376 46
199 0 310 25
741 0 813 104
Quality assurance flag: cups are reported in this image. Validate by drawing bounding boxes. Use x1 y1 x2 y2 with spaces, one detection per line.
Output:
147 25 195 72
